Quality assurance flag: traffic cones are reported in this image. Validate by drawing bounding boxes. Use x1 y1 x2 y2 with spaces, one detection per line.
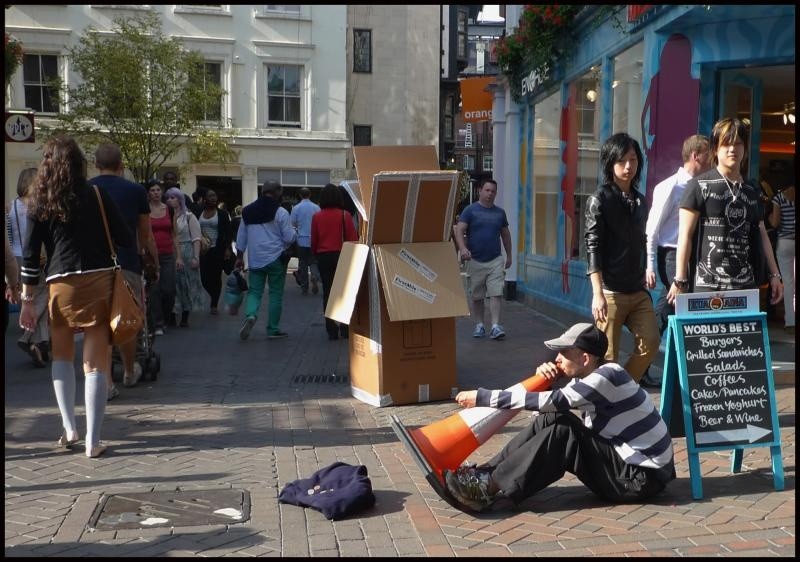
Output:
388 367 561 498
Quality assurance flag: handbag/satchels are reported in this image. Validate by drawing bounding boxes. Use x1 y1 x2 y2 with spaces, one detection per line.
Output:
201 233 210 254
223 267 247 316
111 267 145 346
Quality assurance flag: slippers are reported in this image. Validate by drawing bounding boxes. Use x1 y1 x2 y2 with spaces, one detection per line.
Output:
55 431 80 446
87 443 106 457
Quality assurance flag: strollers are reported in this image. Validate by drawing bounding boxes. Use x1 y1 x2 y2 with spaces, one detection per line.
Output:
109 263 162 382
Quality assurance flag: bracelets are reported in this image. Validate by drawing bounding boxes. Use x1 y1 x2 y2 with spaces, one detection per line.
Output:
769 272 781 280
20 293 34 303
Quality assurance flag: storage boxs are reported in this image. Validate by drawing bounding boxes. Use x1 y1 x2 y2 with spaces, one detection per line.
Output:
324 238 471 410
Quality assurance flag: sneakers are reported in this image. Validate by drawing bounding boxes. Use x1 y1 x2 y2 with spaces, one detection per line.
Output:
473 323 486 339
107 385 121 401
489 324 506 338
268 332 288 338
447 470 500 510
239 316 255 340
123 361 142 386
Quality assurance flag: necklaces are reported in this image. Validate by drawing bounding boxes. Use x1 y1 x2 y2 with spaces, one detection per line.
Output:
720 167 744 204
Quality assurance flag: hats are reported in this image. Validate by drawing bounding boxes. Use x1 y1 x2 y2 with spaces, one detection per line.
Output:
544 323 609 356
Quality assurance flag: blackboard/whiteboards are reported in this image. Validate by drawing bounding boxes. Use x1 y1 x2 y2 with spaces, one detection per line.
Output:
659 311 781 452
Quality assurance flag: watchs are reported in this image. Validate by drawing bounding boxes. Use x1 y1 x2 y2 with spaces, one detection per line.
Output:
671 276 689 290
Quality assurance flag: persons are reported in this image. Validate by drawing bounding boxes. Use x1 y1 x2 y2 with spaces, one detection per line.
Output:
644 134 714 390
235 180 296 342
584 132 659 385
18 134 119 457
138 172 244 336
2 167 50 367
454 179 513 338
284 183 361 339
443 321 677 510
665 115 784 305
747 175 797 335
87 145 160 401
5 230 21 306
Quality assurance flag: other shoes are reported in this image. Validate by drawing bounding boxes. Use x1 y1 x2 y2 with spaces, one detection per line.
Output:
311 277 319 294
30 343 49 369
301 285 309 296
327 323 339 340
178 311 190 327
210 305 218 315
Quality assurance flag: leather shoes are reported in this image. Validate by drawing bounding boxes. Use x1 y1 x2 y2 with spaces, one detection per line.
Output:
640 367 662 389
